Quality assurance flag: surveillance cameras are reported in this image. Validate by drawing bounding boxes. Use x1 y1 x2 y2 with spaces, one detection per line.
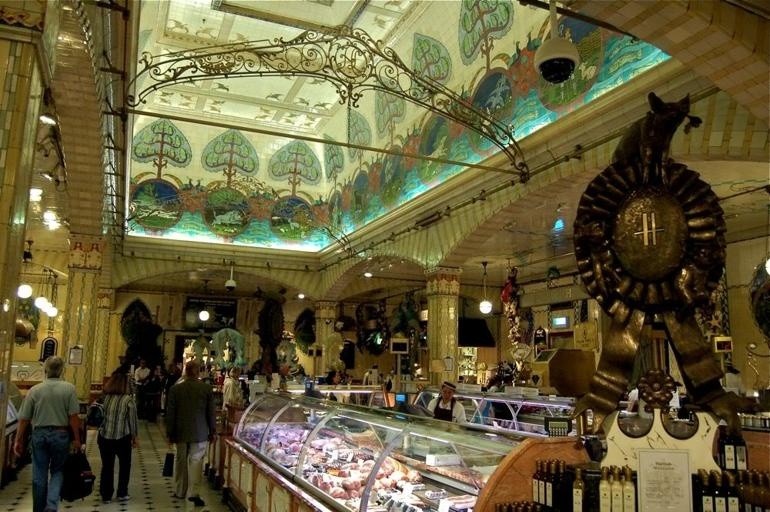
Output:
225 265 237 291
534 0 578 83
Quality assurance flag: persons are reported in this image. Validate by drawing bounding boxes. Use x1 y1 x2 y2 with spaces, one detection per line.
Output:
222 367 244 411
14 356 81 512
134 360 177 423
325 370 341 384
428 381 467 423
97 374 139 504
166 361 217 506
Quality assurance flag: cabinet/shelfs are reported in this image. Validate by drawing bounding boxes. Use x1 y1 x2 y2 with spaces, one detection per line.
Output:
266 383 390 409
735 423 770 476
222 392 592 512
412 389 583 437
3 382 35 484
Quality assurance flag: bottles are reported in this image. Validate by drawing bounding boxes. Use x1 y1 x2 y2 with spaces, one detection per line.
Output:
739 414 769 428
754 474 766 512
599 467 612 512
727 477 739 512
545 463 558 508
737 471 745 512
572 468 585 512
538 462 548 505
717 425 726 467
611 468 623 512
715 476 726 512
700 475 715 512
720 427 736 470
623 467 635 512
532 460 542 503
559 463 566 481
743 473 754 512
736 433 747 470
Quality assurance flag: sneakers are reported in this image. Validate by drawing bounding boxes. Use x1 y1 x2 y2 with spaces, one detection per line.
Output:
188 494 205 507
174 494 185 499
102 498 112 504
118 495 132 501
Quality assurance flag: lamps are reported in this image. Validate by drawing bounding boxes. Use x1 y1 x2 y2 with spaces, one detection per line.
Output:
18 258 32 298
35 270 61 317
480 263 492 314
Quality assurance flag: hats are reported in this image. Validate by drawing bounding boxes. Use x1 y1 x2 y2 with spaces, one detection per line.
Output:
444 381 456 390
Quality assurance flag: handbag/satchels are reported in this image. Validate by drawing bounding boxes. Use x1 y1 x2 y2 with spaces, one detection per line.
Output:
163 447 174 477
59 445 96 503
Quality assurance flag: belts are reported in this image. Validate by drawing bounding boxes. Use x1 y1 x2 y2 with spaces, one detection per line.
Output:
34 425 68 431
